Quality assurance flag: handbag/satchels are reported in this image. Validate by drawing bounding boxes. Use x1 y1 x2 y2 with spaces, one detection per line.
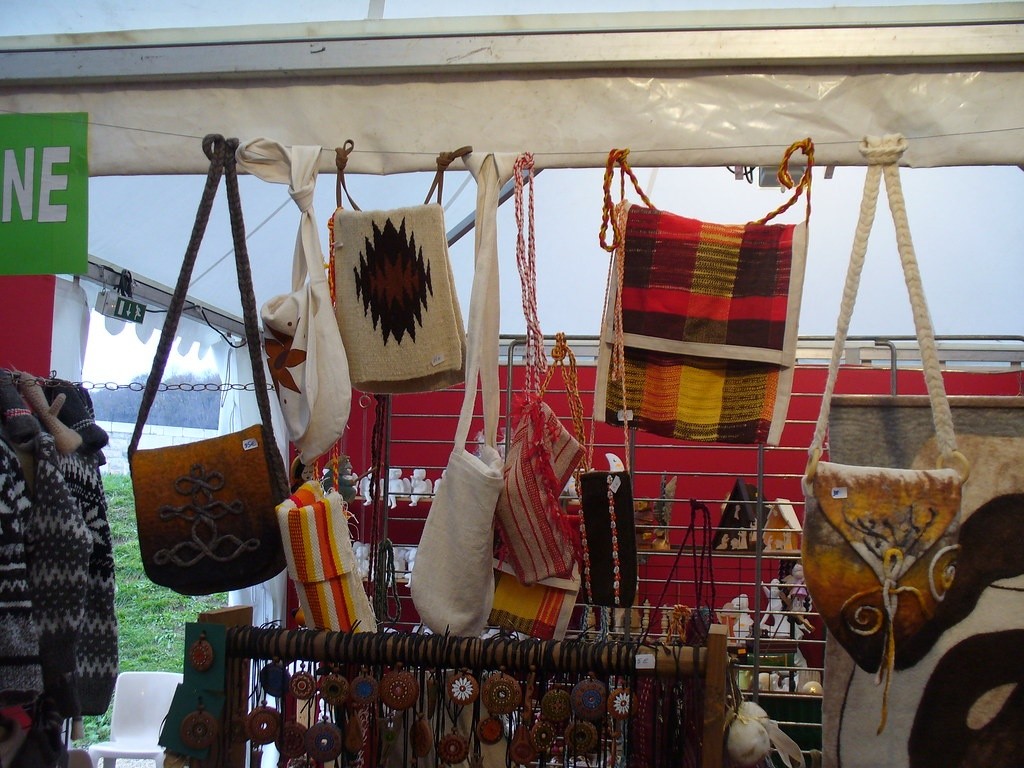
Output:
495 333 592 582
597 140 813 444
801 136 964 736
236 140 352 464
411 444 504 638
129 423 287 596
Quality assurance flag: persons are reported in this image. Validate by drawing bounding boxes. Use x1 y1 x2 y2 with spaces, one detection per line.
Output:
718 564 815 693
353 466 442 587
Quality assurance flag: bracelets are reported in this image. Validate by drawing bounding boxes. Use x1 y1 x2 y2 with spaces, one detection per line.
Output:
581 605 713 646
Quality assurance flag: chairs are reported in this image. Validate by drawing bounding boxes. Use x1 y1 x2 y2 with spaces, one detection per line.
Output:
88 672 184 768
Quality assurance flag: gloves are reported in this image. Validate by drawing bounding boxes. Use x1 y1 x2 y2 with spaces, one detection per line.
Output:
0 370 109 466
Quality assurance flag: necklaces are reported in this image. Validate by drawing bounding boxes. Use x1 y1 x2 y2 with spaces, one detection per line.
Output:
573 472 620 606
178 618 708 768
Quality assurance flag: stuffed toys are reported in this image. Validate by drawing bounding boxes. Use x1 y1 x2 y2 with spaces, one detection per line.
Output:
288 452 315 495
321 455 357 503
728 700 806 768
